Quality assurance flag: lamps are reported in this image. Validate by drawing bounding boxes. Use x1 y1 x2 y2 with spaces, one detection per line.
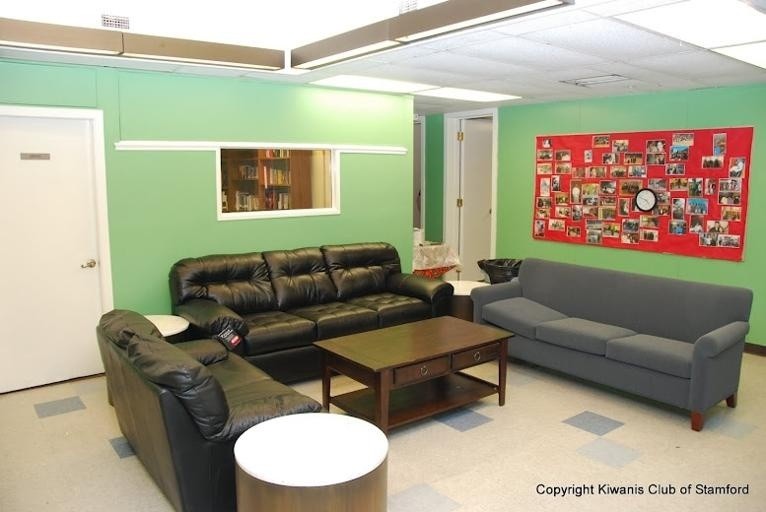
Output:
117 32 284 70
389 0 575 44
0 18 124 56
291 19 408 70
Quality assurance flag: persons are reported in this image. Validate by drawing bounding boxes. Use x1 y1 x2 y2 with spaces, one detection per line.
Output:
533 133 747 249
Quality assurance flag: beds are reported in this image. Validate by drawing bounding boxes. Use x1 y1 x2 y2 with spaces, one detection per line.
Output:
445 280 491 322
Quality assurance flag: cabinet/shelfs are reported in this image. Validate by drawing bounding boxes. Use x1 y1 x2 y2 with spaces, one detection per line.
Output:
222 150 312 213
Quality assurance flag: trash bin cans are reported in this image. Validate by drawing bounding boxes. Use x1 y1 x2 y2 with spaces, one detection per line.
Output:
477 259 523 285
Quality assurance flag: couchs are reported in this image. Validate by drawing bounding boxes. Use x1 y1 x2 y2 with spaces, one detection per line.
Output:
471 258 753 432
97 309 322 512
169 243 453 384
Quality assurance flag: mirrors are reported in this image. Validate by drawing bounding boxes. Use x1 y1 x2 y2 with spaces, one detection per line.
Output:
215 142 341 221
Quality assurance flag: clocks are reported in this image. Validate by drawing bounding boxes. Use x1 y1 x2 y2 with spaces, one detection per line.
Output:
635 188 657 212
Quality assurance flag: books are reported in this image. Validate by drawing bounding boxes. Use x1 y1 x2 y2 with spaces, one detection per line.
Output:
221 149 290 211
